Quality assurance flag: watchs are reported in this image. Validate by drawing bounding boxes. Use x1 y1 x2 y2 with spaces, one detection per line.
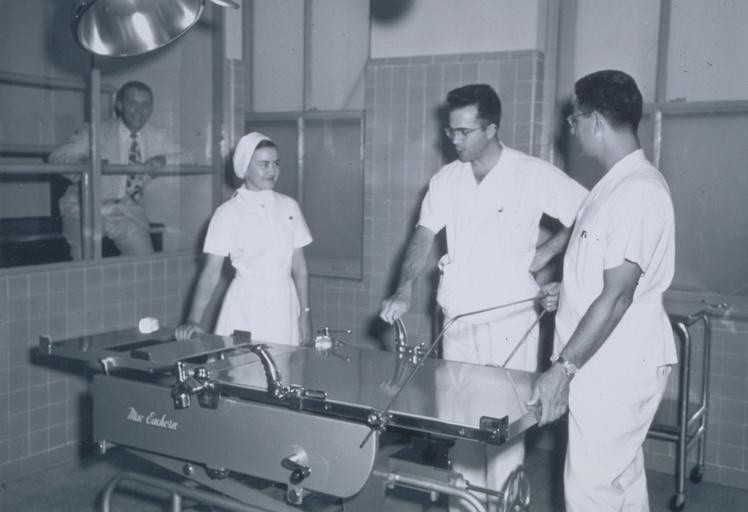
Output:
556 357 579 379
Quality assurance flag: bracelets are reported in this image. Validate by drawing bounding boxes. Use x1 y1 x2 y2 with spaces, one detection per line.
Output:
300 307 312 315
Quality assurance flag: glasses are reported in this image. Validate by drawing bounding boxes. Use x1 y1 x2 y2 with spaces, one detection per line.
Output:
566 112 590 128
443 124 481 139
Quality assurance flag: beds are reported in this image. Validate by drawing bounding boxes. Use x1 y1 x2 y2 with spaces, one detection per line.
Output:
32 324 564 512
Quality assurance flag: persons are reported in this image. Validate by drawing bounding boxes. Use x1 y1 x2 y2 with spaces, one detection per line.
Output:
174 132 316 345
527 70 675 511
46 82 194 260
377 84 591 512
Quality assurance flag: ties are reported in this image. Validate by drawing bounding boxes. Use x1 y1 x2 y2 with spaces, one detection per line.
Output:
125 133 144 205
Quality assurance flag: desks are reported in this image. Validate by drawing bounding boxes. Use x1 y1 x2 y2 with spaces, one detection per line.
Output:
647 298 712 508
